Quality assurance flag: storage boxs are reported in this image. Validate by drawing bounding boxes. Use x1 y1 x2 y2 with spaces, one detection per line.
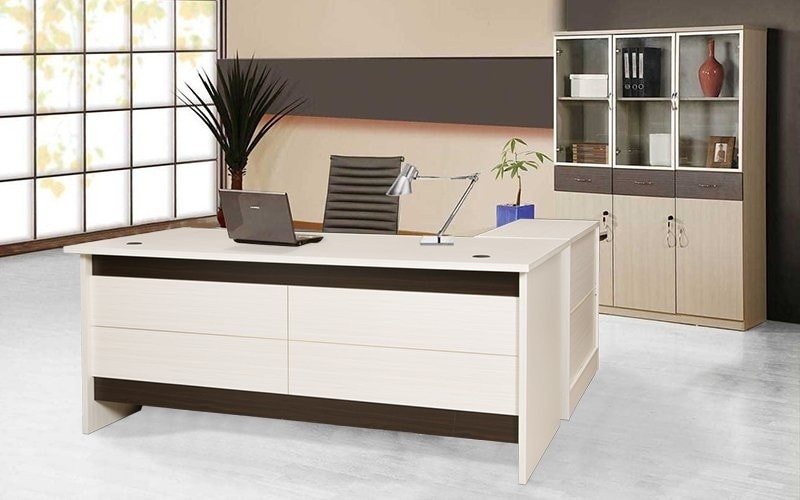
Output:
568 73 608 99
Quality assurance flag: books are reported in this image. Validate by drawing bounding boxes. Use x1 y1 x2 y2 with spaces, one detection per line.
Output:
573 143 608 164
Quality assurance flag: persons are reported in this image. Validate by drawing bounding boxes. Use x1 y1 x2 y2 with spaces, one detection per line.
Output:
717 144 725 162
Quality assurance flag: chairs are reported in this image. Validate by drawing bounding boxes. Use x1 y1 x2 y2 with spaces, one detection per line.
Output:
325 157 407 237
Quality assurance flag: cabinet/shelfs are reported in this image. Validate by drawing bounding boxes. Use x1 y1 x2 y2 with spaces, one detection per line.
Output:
551 23 766 331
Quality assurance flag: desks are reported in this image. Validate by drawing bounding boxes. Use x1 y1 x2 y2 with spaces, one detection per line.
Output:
61 217 599 486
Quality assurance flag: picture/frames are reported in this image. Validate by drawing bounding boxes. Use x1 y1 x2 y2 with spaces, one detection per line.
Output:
705 136 735 170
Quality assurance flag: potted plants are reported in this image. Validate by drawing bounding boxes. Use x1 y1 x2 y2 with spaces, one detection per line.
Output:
172 50 308 229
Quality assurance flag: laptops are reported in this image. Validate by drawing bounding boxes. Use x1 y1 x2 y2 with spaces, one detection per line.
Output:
218 189 323 247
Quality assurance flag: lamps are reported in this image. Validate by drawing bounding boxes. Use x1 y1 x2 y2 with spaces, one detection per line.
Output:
385 164 481 245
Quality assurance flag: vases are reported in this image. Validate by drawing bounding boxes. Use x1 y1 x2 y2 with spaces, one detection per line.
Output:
700 39 725 99
492 138 554 227
